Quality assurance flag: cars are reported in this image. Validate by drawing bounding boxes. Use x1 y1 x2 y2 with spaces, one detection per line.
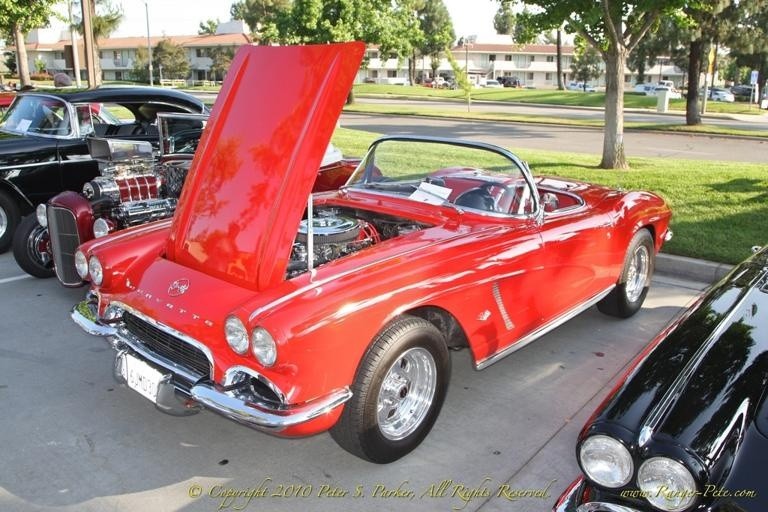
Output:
69 42 676 465
634 80 768 104
550 244 767 512
565 82 595 92
416 71 521 89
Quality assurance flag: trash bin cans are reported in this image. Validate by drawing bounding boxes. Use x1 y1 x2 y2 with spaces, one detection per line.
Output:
656 87 671 113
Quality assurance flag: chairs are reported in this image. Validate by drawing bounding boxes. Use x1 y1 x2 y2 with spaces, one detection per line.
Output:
443 175 584 215
94 124 140 136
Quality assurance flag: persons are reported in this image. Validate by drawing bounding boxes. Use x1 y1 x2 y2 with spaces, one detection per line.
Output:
53 72 72 87
